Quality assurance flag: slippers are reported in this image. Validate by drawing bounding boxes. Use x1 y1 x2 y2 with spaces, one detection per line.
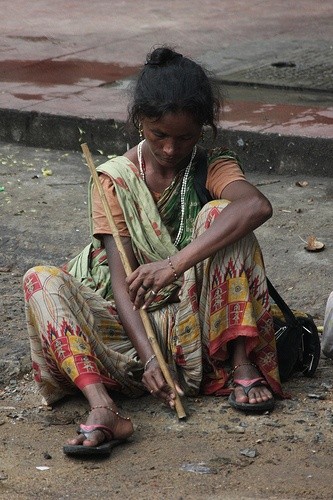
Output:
229 376 277 411
64 424 127 457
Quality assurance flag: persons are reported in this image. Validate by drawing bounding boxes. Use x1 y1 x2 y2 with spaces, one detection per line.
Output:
22 44 292 458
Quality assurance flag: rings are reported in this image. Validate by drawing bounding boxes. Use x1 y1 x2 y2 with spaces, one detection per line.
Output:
140 283 149 290
151 289 157 296
150 389 154 395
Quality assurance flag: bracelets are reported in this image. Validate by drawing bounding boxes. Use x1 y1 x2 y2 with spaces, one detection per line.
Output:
144 353 158 372
166 256 181 279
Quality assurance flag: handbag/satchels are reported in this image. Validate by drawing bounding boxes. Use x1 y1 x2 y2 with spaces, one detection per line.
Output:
271 315 321 383
